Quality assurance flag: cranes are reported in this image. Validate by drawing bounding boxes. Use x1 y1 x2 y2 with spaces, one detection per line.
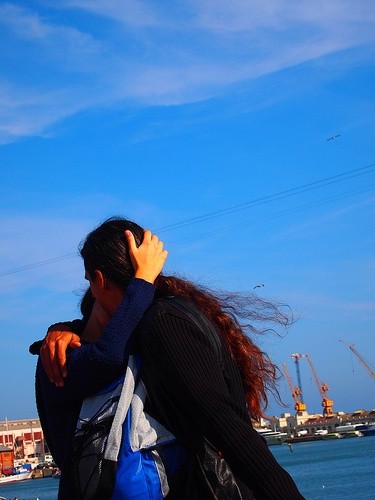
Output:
300 354 334 415
281 362 306 411
338 339 375 381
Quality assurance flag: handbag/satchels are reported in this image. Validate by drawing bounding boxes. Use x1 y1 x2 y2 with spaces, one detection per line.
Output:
161 429 242 500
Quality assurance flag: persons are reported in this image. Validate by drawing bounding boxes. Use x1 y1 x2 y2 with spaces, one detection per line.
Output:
35 230 176 500
28 215 308 500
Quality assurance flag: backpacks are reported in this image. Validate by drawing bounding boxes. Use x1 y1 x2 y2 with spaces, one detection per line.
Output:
67 355 170 500
